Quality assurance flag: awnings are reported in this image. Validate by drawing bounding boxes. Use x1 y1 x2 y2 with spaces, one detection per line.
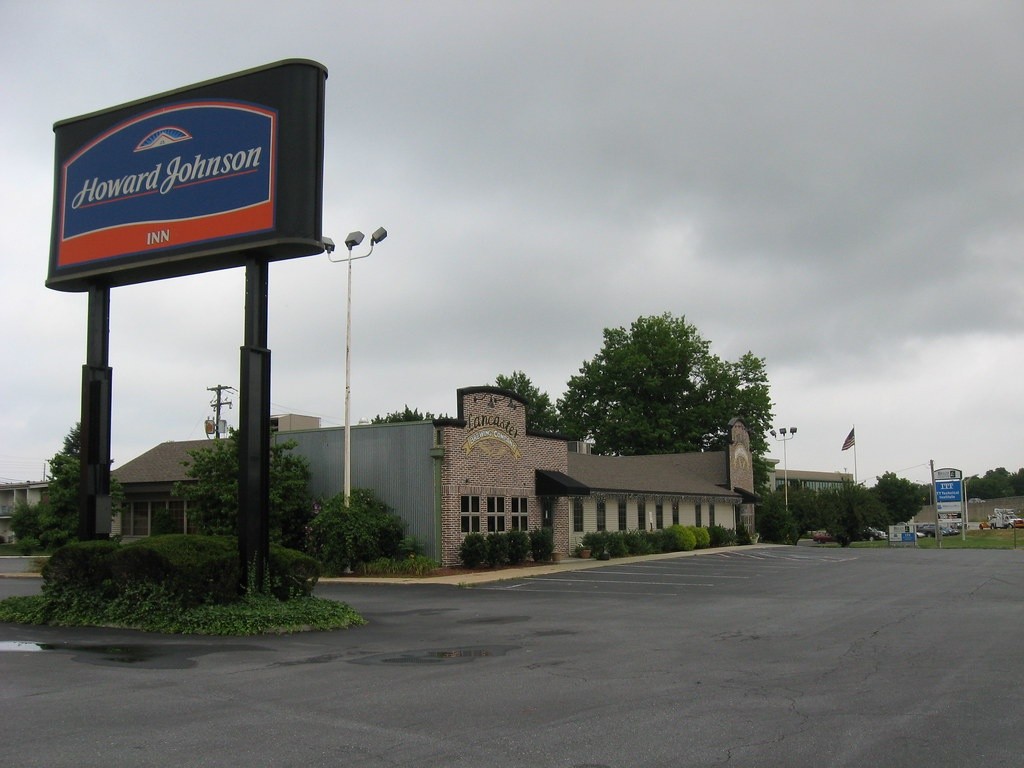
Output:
534 469 590 497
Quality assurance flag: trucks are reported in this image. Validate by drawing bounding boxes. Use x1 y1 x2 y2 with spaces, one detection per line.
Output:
979 507 1024 530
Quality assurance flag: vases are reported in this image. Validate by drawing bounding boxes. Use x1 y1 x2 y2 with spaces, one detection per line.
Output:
552 552 563 561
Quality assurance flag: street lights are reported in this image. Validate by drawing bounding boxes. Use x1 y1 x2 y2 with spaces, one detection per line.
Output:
322 227 389 573
769 427 798 513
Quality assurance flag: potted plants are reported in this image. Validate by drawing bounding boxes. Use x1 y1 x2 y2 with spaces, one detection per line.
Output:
575 532 592 559
749 533 760 545
597 529 611 560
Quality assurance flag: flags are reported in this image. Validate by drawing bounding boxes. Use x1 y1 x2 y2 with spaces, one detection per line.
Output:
841 428 854 452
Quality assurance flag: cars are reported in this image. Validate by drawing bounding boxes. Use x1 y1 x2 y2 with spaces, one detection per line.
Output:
915 522 960 538
863 528 888 541
812 531 836 544
